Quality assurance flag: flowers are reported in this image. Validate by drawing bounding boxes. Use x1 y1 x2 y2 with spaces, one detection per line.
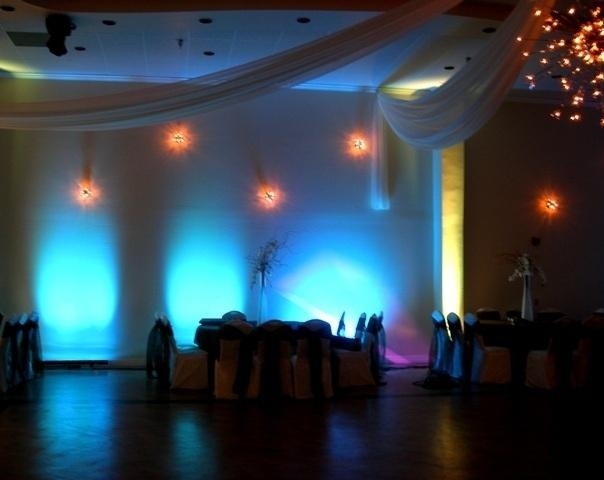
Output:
506 253 546 285
244 229 291 290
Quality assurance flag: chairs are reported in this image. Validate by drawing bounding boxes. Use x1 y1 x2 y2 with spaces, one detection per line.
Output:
0 309 47 395
429 306 604 401
144 308 388 403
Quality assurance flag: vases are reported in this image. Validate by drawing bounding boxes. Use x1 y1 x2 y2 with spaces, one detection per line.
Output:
254 271 269 324
519 273 535 321
